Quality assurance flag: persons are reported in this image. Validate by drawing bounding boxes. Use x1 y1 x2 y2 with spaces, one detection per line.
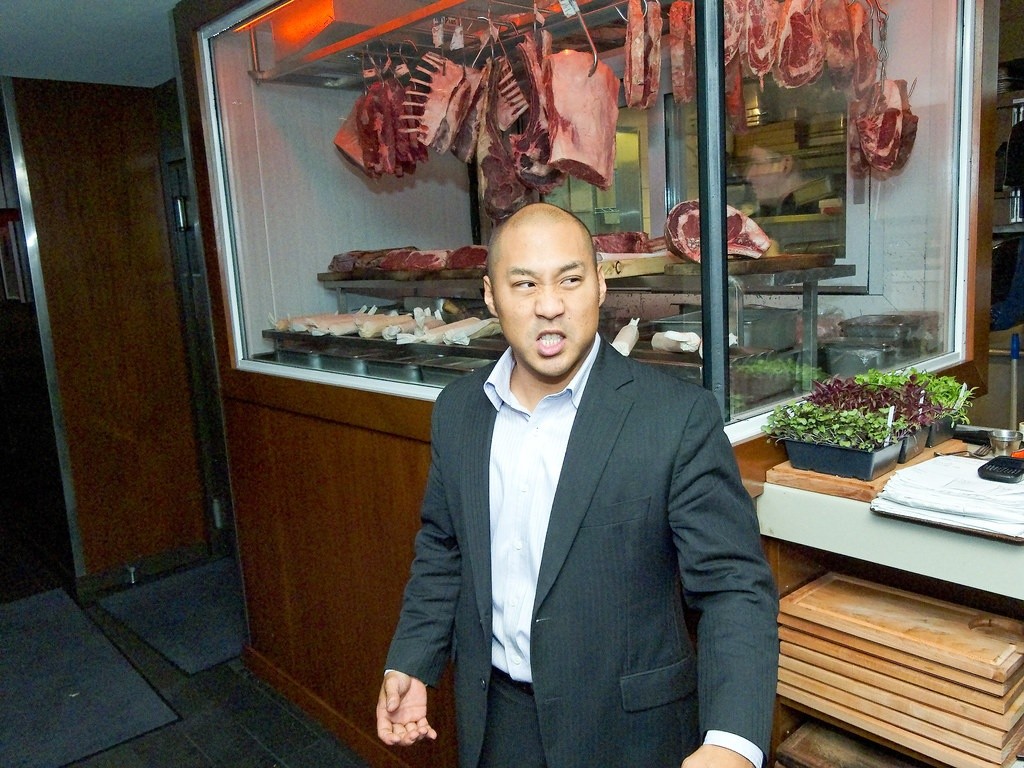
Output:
744 134 847 217
373 203 778 768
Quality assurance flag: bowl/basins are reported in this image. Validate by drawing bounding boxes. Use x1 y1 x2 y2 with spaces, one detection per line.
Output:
987 430 1023 457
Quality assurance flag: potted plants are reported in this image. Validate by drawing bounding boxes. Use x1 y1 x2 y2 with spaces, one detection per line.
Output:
758 366 981 483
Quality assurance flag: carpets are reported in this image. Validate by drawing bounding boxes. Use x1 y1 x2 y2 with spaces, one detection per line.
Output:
0 588 182 768
96 553 246 676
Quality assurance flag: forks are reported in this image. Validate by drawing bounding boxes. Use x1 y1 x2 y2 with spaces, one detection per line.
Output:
934 444 993 458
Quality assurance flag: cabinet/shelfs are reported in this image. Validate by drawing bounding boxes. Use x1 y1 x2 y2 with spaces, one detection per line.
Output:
754 485 1024 768
